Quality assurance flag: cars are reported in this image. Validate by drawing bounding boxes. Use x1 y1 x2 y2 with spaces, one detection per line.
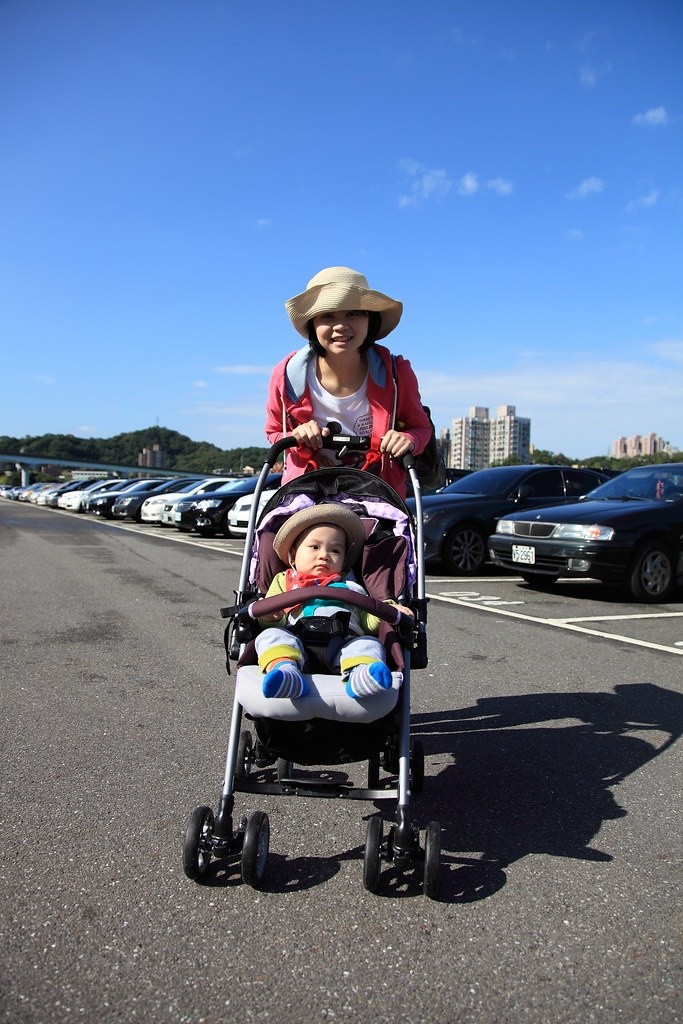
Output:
404 465 615 576
487 462 683 604
227 488 289 537
175 473 284 536
0 478 239 525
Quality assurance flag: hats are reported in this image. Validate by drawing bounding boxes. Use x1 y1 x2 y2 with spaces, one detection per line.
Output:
272 503 366 567
284 266 404 341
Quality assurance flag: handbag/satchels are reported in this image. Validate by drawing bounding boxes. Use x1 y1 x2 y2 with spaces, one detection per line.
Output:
405 405 447 499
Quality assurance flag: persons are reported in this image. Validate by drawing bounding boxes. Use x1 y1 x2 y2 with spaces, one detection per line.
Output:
244 499 394 702
263 265 433 500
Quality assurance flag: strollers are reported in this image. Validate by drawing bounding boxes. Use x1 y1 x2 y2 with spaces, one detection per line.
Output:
184 429 441 899
